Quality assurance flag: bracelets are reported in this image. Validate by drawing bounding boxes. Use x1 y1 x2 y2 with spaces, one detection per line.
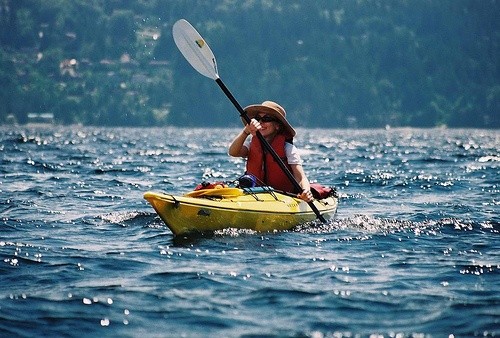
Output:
243 130 249 136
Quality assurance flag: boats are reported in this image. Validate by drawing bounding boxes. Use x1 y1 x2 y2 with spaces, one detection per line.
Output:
144 183 338 238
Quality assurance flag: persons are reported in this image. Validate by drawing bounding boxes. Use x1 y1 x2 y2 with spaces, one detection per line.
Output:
228 100 315 203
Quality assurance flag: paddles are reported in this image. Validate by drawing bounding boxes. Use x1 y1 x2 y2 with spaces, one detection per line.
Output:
187 185 274 197
170 16 330 225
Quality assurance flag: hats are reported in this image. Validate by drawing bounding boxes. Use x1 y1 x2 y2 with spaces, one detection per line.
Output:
242 101 296 140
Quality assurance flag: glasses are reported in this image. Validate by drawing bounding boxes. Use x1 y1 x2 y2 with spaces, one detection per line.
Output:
253 114 272 123
